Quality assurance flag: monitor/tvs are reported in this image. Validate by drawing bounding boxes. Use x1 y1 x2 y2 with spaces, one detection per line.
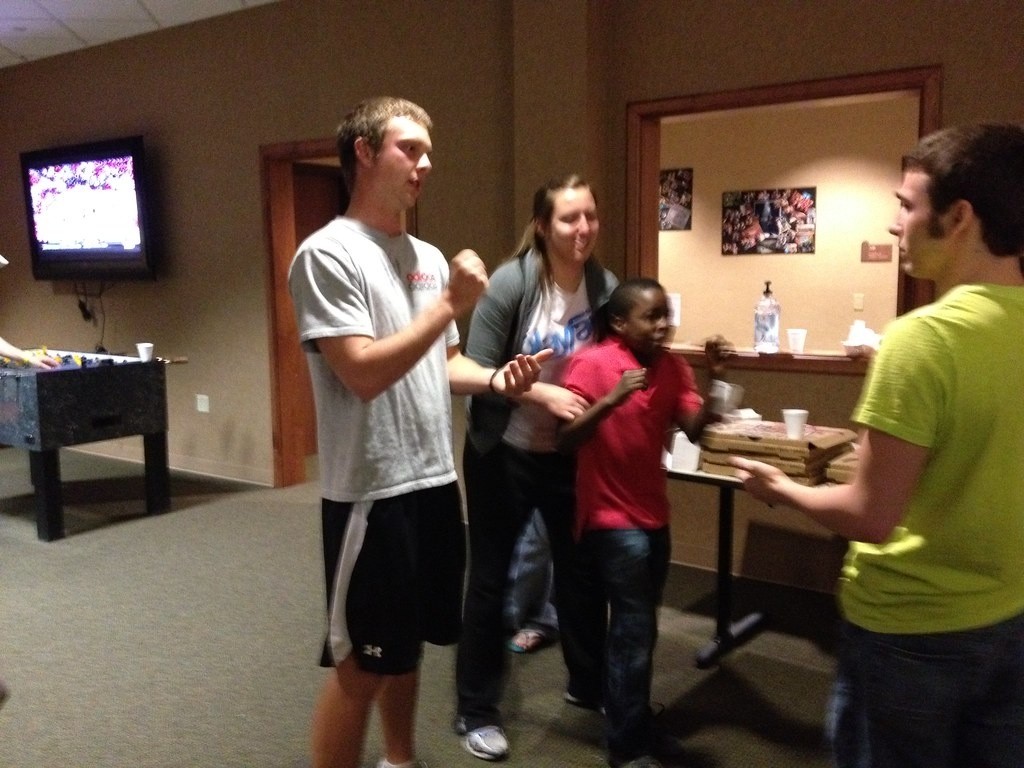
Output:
19 135 158 281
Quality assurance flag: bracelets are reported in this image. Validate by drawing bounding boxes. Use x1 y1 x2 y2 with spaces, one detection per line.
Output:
489 368 499 393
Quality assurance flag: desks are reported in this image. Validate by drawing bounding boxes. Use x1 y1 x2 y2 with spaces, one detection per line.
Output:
0 348 170 541
661 468 841 666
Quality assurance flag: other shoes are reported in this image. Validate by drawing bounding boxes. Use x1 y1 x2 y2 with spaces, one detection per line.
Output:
454 711 511 761
507 626 544 654
564 691 590 705
607 751 664 768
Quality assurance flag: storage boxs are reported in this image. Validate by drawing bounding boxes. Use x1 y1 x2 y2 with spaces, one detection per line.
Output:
700 421 859 487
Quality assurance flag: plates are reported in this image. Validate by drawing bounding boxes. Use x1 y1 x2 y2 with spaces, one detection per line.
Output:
839 341 881 357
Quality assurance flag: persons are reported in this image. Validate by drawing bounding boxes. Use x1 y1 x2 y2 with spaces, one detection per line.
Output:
725 121 1024 768
288 97 553 768
0 337 56 369
453 170 736 761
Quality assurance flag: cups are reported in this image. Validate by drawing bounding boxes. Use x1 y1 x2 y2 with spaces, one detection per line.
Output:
781 408 809 440
135 342 155 363
786 328 807 351
